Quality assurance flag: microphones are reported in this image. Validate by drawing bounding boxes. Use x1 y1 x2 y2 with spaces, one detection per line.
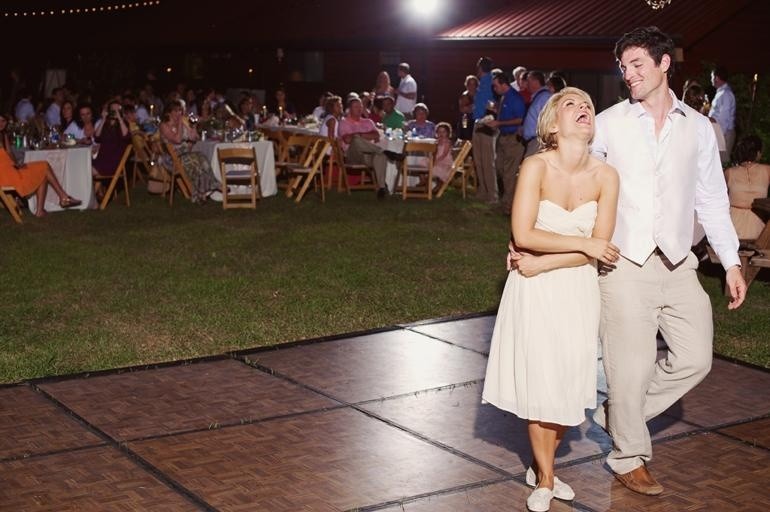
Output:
752 72 760 107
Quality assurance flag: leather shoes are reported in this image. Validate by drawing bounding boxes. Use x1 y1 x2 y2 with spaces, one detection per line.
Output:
611 465 664 495
377 188 386 201
390 150 405 162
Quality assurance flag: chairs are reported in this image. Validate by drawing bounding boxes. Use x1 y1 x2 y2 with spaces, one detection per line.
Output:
705 196 770 297
1 118 479 225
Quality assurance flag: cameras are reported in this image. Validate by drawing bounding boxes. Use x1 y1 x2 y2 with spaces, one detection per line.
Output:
108 109 117 117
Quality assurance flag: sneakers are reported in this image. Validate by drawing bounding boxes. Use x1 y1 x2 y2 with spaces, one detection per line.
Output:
526 483 554 512
526 465 575 501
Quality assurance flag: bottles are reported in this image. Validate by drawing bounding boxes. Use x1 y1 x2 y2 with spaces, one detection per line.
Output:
187 111 263 142
4 119 79 152
374 125 436 145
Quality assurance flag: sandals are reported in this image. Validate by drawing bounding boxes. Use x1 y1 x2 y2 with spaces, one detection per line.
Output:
35 209 47 219
60 196 82 208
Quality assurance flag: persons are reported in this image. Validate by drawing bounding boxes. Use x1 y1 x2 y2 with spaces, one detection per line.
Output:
455 57 566 207
1 85 296 214
719 130 770 245
313 62 454 200
481 87 620 511
681 65 734 172
506 25 747 496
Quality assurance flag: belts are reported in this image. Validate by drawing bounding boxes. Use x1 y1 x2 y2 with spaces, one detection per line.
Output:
653 248 663 256
